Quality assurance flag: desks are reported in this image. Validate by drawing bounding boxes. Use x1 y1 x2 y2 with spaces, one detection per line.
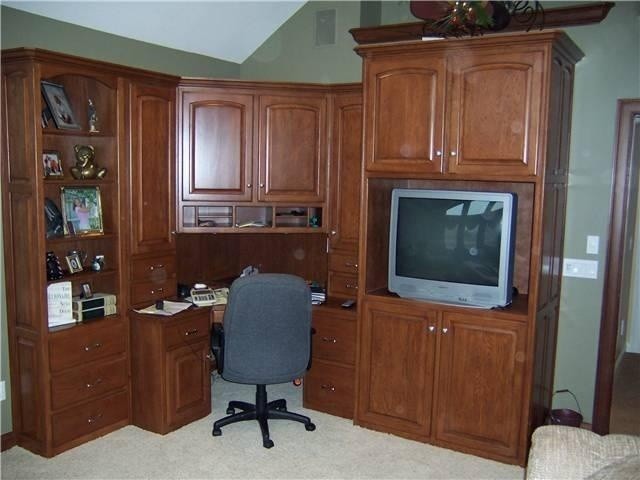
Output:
130 294 358 440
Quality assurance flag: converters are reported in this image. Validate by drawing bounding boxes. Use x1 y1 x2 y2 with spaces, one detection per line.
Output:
156 300 163 309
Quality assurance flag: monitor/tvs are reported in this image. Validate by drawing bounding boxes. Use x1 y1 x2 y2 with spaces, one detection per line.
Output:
387 188 518 309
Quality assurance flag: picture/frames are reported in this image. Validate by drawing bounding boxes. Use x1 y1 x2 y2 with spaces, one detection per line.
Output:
43 149 65 180
59 184 106 238
41 80 81 131
63 254 84 274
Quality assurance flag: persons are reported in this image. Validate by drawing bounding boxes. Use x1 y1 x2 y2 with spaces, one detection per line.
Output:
86 96 98 133
71 196 93 231
53 93 72 124
44 155 62 175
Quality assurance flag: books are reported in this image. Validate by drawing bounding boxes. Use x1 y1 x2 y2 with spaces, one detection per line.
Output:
311 285 328 305
72 304 116 322
71 292 117 311
238 220 267 228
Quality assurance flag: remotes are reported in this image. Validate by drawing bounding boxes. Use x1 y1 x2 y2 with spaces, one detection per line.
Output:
342 300 355 307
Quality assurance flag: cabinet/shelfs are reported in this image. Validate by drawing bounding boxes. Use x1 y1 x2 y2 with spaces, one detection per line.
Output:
128 65 178 305
175 76 333 232
1 45 132 459
367 53 543 180
366 177 534 316
362 305 530 463
328 81 363 306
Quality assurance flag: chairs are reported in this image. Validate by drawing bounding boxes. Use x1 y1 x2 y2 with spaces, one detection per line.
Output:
209 272 316 448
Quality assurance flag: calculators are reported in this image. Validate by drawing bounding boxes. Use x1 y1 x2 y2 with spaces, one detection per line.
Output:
190 284 217 307
